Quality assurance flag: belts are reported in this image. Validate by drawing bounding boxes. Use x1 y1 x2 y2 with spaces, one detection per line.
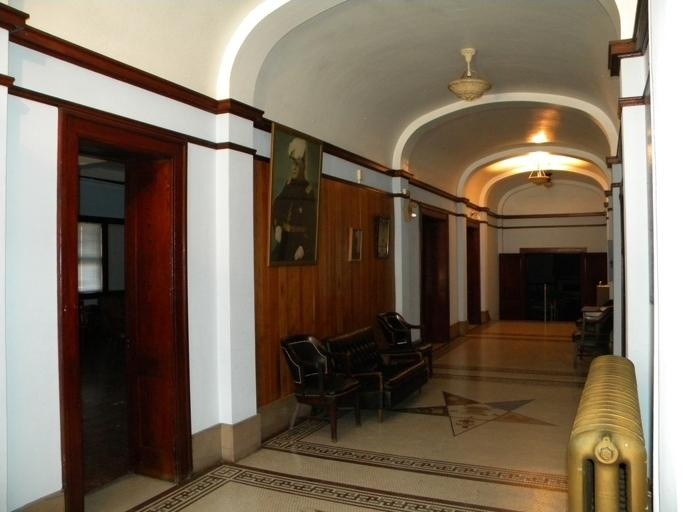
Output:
281 224 309 232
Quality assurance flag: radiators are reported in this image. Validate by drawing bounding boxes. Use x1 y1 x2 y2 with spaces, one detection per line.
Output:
567 355 647 512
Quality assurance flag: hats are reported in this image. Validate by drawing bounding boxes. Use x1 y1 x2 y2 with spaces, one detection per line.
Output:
289 150 305 161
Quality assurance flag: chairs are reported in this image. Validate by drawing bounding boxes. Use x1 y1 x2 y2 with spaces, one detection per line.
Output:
280 335 360 442
326 326 428 416
572 310 613 360
576 300 613 326
375 312 432 377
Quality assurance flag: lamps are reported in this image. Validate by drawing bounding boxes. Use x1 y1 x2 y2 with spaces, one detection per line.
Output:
528 163 551 185
447 49 491 100
407 202 417 223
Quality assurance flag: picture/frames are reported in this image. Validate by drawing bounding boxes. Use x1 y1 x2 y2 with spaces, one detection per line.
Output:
348 228 362 262
375 216 391 259
267 122 323 266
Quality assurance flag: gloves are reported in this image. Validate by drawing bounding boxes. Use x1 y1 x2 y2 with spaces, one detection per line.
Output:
275 226 282 242
294 246 305 260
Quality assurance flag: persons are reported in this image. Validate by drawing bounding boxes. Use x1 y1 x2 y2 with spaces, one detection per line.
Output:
271 137 316 261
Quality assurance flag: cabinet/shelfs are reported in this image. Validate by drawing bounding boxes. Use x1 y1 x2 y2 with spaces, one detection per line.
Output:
596 285 611 306
